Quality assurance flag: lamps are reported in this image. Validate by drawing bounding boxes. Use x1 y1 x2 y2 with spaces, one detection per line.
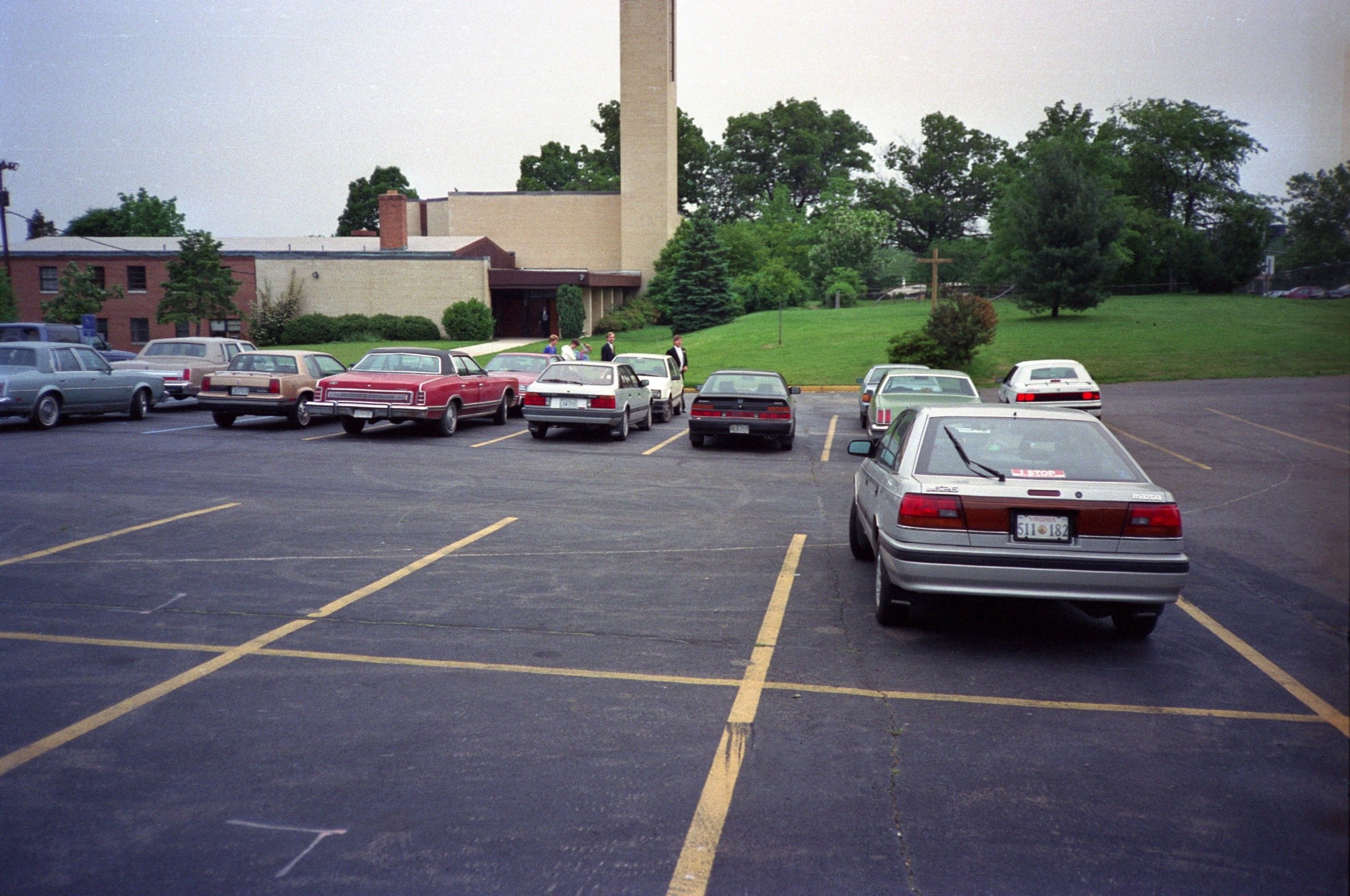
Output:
579 274 585 281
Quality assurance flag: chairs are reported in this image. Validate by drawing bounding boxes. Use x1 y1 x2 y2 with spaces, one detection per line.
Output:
652 365 664 375
185 347 199 356
719 381 737 392
757 383 774 393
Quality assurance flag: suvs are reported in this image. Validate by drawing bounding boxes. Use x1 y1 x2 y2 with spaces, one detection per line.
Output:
0 322 138 363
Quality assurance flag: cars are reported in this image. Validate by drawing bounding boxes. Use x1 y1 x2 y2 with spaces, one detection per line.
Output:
1328 284 1350 300
865 369 990 454
483 352 566 418
0 341 170 431
196 349 355 431
688 370 801 451
995 359 1102 421
521 360 654 442
305 347 523 438
847 402 1191 638
1277 286 1327 299
600 353 684 423
107 338 274 401
1263 290 1287 299
856 364 942 429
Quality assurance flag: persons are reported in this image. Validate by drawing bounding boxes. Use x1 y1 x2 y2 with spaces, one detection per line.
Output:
600 331 616 363
575 342 592 361
561 338 581 361
667 334 688 382
538 306 550 339
544 334 559 355
